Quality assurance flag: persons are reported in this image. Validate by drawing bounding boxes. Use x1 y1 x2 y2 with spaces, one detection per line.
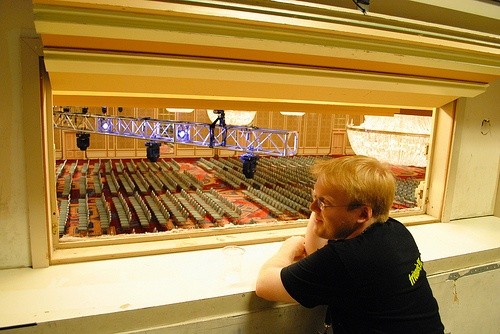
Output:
256 155 444 334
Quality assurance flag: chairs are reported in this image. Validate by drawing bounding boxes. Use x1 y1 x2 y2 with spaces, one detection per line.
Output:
55 155 421 235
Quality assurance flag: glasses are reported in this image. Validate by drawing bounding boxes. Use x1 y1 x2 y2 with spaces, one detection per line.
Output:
312 191 366 210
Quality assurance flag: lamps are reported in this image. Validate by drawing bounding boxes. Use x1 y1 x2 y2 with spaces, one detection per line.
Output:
346 114 432 167
206 109 257 127
